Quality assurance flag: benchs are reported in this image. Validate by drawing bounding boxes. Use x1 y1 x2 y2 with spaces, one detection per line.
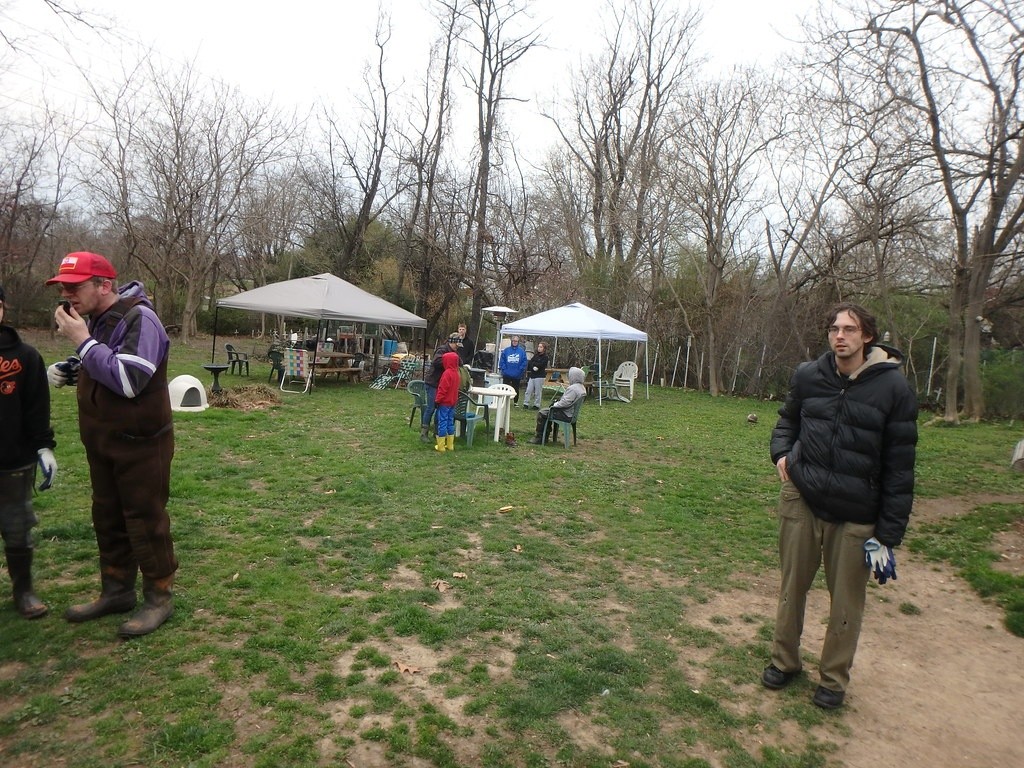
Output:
309 362 361 373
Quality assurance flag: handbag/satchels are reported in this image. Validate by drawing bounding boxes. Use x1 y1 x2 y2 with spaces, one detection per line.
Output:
458 365 471 393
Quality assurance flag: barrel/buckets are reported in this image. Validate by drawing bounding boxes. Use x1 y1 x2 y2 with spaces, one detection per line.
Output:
484 372 503 404
470 367 486 399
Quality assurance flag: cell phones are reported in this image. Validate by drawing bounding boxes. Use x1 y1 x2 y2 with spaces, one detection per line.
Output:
59 300 72 317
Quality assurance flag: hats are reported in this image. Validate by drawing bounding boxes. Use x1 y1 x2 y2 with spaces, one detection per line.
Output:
0 285 5 301
448 337 464 347
512 336 518 342
45 251 117 282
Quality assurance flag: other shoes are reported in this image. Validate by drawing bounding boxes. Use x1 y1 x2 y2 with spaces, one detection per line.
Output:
531 406 538 411
514 403 519 408
522 406 528 410
527 440 536 444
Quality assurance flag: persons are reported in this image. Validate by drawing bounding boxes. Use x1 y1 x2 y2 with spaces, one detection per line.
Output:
421 325 475 452
760 303 919 710
499 336 527 406
532 367 586 443
43 252 178 634
524 343 548 410
0 289 57 618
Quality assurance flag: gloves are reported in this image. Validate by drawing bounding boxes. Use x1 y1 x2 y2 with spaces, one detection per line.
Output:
532 366 538 372
46 361 78 388
864 537 897 586
37 447 57 492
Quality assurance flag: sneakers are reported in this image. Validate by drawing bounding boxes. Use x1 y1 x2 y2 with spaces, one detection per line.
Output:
762 663 794 689
812 685 845 708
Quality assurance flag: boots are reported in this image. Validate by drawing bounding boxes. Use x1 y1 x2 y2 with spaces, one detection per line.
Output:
420 424 431 444
64 558 139 623
433 424 439 438
493 427 504 441
434 437 445 452
445 433 454 452
4 547 48 619
506 430 521 447
117 570 175 637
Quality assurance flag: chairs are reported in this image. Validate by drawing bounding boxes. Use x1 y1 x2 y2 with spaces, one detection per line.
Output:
612 361 639 401
280 347 313 394
225 344 249 377
455 390 489 447
474 384 516 429
368 354 417 390
407 380 428 427
268 351 284 383
542 395 587 448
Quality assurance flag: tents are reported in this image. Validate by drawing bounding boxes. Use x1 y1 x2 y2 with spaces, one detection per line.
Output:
497 302 649 407
212 273 428 394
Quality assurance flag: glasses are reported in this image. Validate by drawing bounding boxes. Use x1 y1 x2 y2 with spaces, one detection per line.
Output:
55 280 101 297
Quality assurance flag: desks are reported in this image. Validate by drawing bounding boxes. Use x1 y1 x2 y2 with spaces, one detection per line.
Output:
309 353 356 386
456 387 517 442
